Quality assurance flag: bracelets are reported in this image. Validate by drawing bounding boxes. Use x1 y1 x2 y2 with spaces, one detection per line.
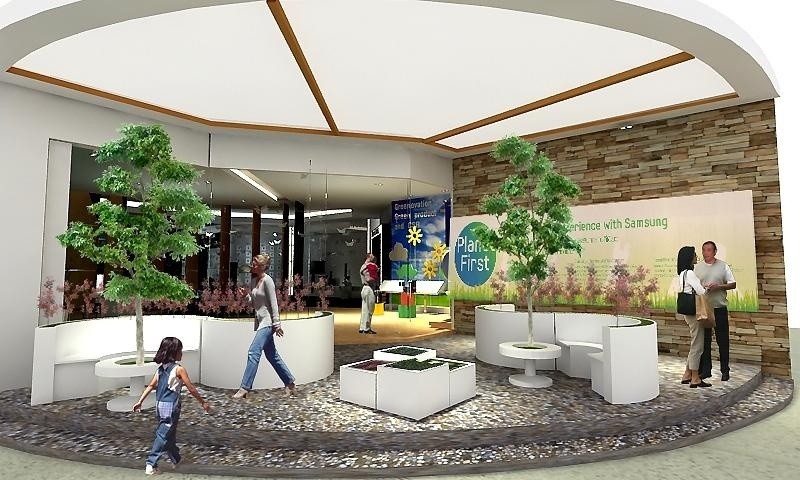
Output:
201 399 205 408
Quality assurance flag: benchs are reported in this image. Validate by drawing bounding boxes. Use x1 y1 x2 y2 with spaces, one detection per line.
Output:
554 338 617 401
54 339 201 401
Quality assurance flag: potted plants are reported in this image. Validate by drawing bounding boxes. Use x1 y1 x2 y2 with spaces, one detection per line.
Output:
339 345 478 422
469 133 585 389
55 118 214 416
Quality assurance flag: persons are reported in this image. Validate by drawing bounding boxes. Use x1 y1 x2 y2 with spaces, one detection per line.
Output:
693 241 737 381
363 255 380 285
676 245 714 387
230 253 298 402
358 253 381 335
131 336 211 475
